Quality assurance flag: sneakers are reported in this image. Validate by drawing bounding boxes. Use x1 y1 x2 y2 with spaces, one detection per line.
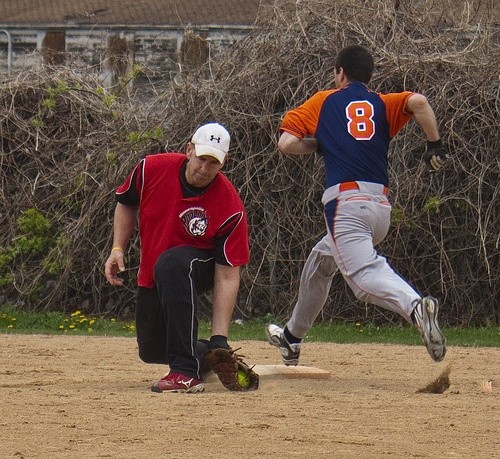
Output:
153 373 204 393
268 323 300 366
415 297 446 361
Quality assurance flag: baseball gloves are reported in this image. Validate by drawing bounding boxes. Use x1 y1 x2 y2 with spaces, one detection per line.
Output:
206 334 260 392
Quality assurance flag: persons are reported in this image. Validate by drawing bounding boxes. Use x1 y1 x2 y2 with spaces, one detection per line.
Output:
105 124 259 392
266 45 450 366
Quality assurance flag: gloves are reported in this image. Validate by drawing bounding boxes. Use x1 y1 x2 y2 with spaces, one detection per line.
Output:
424 140 448 172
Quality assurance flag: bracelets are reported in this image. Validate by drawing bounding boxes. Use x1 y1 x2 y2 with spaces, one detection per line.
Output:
111 247 124 253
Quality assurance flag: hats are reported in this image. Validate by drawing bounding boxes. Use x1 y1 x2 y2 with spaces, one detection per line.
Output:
191 123 230 164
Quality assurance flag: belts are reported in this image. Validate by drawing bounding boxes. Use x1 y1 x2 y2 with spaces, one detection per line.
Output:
340 182 389 196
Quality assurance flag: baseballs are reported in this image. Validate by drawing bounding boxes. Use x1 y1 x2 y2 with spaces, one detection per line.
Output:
235 369 251 388
